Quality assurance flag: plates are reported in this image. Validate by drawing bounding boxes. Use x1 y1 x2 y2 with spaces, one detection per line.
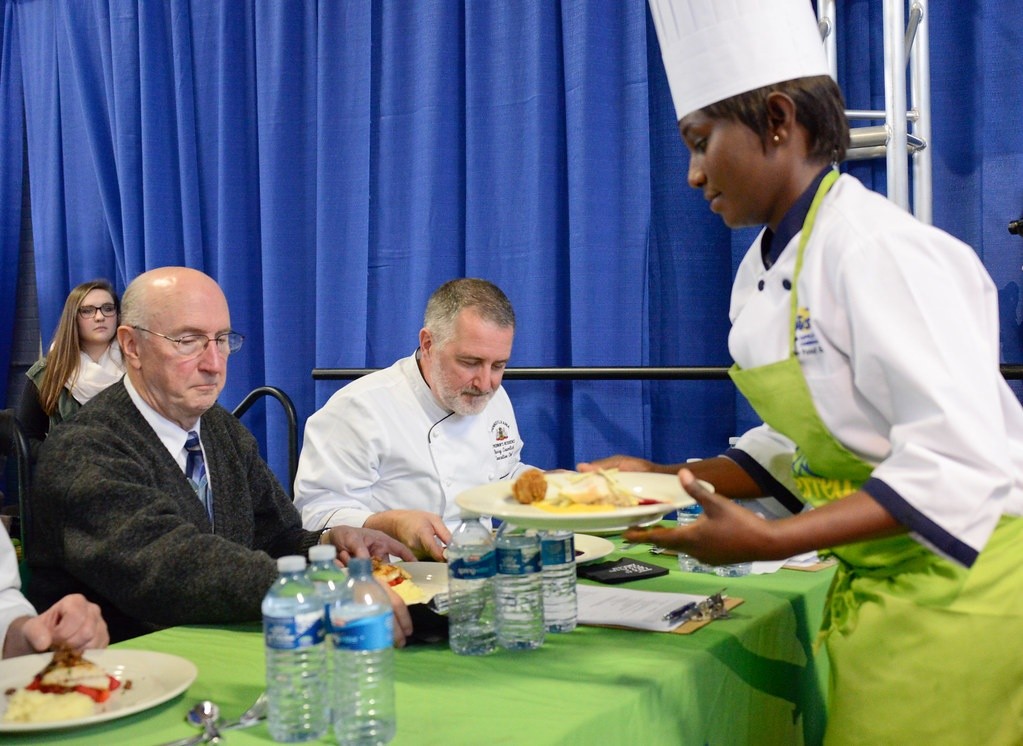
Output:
574 532 616 565
0 648 199 733
576 514 665 536
455 471 715 532
390 561 477 604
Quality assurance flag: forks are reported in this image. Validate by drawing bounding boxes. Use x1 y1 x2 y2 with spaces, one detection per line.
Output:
425 589 468 614
153 689 268 746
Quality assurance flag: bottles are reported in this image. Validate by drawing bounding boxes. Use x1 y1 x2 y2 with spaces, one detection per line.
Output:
494 520 545 652
446 508 500 658
677 457 752 578
260 556 329 741
302 544 347 724
330 556 396 746
537 529 578 633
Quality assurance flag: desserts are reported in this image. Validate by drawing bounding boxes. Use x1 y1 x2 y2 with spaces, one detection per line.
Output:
5 653 122 724
370 564 426 604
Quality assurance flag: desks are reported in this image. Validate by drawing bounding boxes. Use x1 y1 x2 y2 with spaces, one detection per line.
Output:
0 519 840 746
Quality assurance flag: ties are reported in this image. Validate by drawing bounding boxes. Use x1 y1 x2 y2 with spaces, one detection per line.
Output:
182 431 214 530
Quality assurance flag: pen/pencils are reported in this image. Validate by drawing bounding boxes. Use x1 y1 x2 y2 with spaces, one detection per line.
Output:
664 602 696 620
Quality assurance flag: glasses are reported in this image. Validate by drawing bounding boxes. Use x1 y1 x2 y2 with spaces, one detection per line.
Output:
131 325 244 360
77 303 119 320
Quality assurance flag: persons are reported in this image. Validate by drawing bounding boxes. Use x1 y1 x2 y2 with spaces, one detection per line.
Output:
293 278 569 564
571 0 1023 746
21 277 129 463
0 517 111 659
22 266 419 650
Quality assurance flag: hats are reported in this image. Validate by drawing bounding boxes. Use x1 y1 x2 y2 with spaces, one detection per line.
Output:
644 0 831 122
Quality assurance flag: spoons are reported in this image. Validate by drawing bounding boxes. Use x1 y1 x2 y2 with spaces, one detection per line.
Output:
187 700 225 744
